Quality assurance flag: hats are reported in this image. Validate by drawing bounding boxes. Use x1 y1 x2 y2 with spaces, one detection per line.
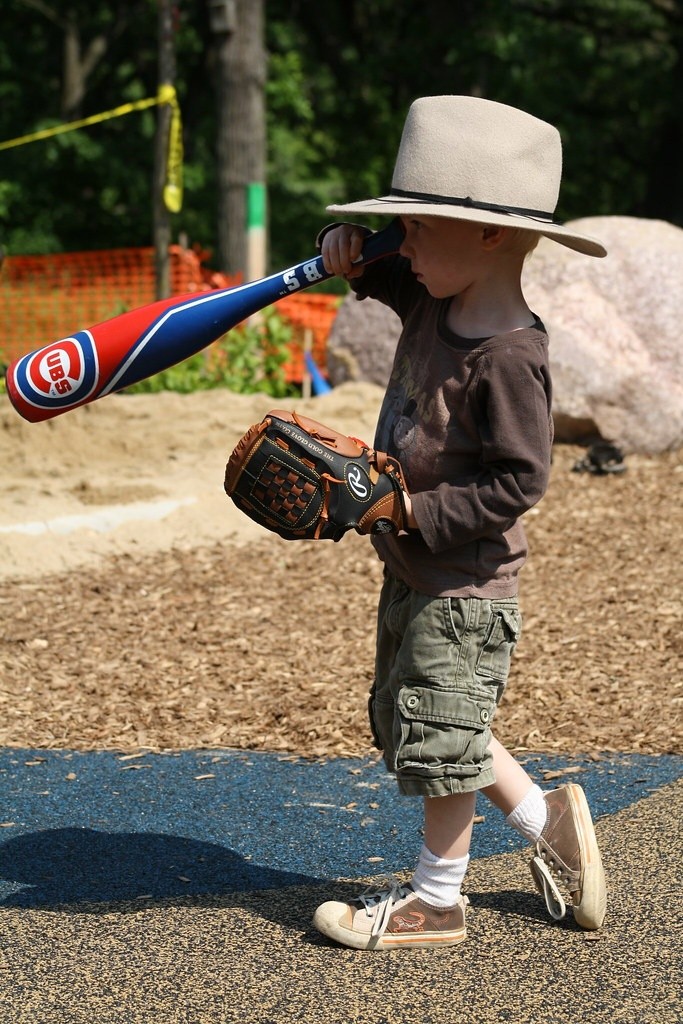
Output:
325 96 609 259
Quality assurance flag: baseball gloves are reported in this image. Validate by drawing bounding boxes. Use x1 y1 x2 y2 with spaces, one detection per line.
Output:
222 409 409 544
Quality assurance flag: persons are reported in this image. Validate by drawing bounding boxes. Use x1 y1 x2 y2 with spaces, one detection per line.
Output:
224 94 608 950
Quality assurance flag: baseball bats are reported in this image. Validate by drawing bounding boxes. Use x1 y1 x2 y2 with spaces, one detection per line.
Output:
4 214 407 425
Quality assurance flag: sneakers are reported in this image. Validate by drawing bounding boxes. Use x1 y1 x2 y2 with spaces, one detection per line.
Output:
530 782 607 929
314 873 470 950
